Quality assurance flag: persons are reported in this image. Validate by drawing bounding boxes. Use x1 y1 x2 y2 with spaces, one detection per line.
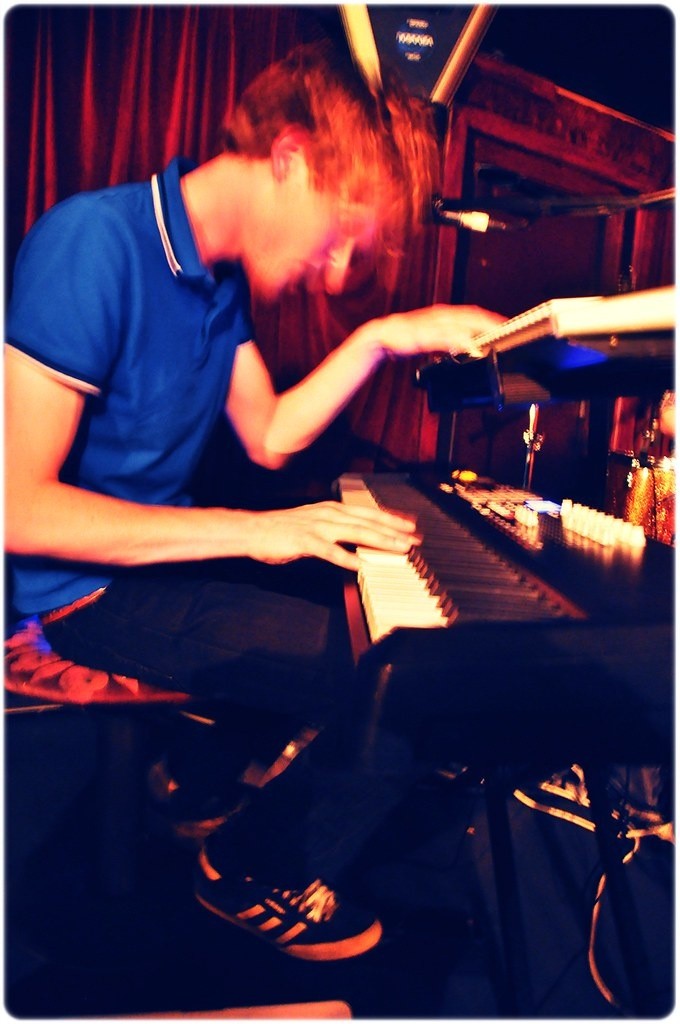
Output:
3 42 504 959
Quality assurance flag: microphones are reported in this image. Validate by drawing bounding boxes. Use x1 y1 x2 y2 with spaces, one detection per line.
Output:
419 193 510 235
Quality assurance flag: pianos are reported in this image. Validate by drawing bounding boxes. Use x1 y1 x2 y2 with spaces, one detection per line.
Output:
325 457 674 768
412 286 676 414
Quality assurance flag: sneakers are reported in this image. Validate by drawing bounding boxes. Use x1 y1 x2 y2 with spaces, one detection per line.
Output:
194 844 384 960
150 764 240 839
